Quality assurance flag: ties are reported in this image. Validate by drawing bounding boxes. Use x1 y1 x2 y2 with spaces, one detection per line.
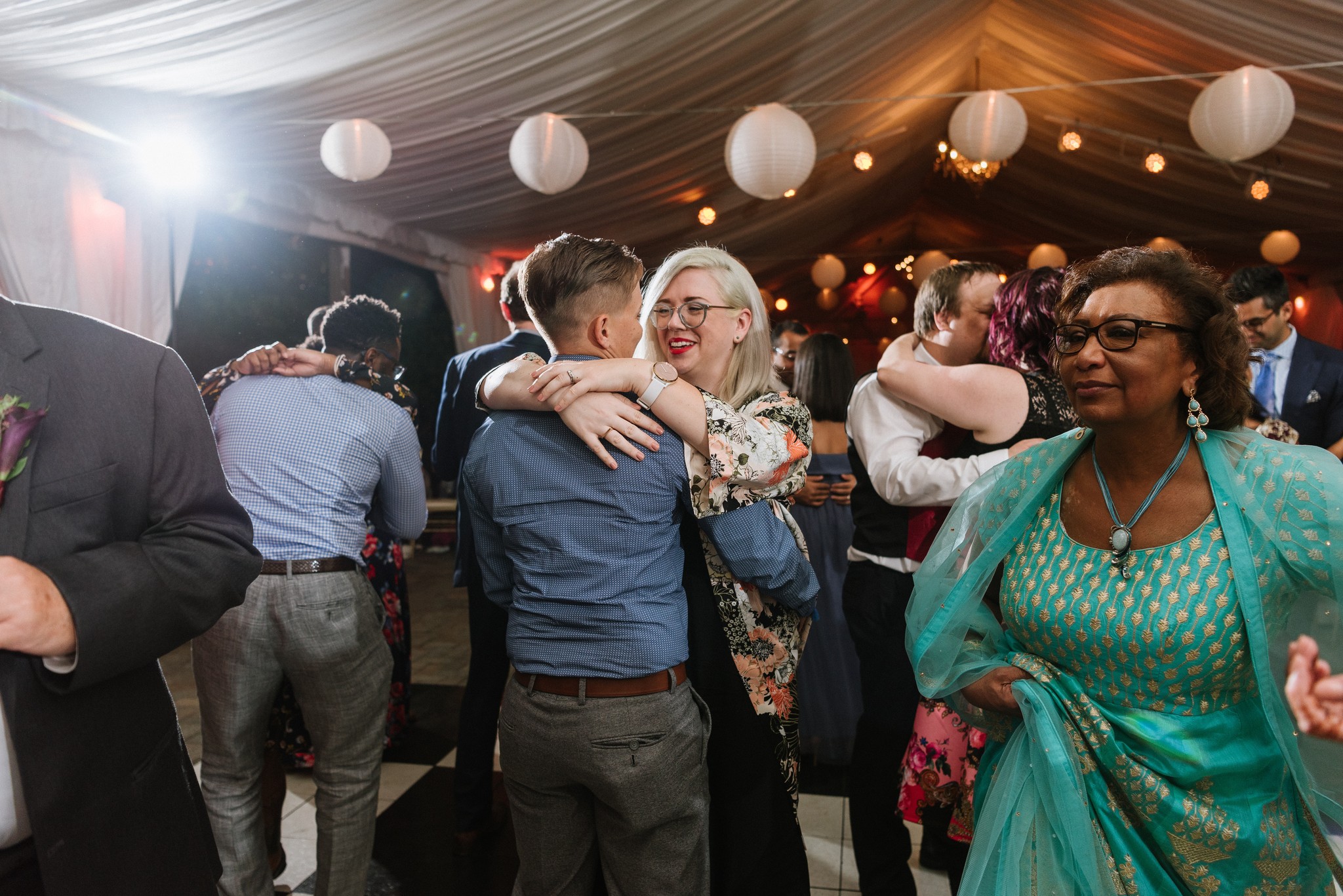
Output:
1254 352 1283 420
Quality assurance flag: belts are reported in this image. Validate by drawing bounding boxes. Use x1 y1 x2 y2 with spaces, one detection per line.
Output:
260 555 357 575
513 661 688 699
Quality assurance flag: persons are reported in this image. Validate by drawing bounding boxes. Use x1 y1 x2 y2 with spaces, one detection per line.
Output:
0 288 263 896
194 230 1343 896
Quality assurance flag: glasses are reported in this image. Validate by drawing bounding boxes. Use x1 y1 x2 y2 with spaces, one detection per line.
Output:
1245 311 1275 333
1051 319 1198 355
649 301 736 329
771 345 796 362
361 348 408 381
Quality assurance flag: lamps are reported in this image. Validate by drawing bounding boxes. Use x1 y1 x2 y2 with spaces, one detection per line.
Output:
932 58 1011 190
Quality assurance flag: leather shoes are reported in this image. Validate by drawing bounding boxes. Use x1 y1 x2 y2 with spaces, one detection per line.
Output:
452 801 507 857
